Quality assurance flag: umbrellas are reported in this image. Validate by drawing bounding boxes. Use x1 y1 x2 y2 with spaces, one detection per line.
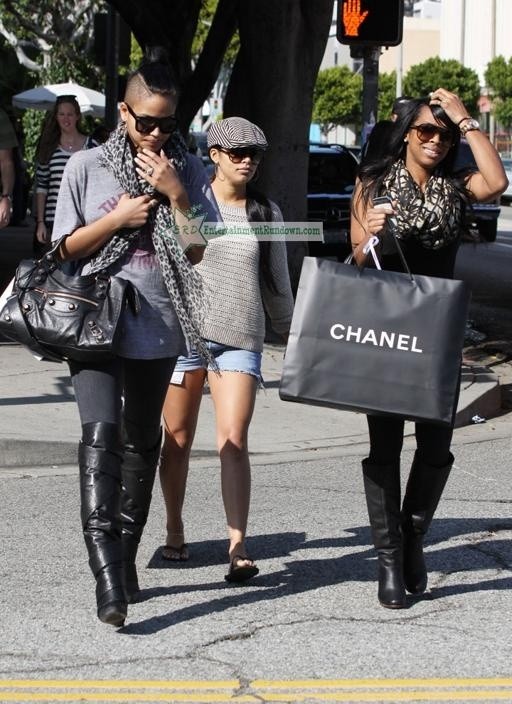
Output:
13 77 113 124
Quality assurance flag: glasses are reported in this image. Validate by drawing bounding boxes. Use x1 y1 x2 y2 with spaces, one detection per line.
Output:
214 144 266 163
409 122 455 148
124 101 182 136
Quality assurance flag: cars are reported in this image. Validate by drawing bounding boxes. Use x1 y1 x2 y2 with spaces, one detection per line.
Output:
191 129 213 156
305 141 511 333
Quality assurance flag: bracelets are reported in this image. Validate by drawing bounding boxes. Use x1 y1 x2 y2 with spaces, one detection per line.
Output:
450 114 475 126
37 220 45 222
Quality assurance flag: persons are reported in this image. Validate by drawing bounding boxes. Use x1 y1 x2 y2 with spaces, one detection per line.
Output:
50 45 224 629
36 95 98 257
346 88 509 612
391 97 415 120
14 78 107 118
0 110 21 230
157 116 297 585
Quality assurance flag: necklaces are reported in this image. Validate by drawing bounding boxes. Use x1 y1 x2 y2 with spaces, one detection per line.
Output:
64 136 79 151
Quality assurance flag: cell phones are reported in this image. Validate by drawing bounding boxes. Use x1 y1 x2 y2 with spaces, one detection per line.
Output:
371 195 399 231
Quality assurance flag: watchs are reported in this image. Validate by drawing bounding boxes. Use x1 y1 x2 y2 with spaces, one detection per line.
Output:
2 191 16 200
460 119 483 135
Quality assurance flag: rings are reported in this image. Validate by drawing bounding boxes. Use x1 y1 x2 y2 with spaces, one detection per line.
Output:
144 164 154 177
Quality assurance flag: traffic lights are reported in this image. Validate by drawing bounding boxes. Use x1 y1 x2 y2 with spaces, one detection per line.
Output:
338 0 402 45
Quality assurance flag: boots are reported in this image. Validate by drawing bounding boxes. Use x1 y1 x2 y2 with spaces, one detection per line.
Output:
77 418 160 627
361 457 408 610
401 449 456 597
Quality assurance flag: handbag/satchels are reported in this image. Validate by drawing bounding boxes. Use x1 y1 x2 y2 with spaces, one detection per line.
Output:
278 215 473 427
0 232 136 364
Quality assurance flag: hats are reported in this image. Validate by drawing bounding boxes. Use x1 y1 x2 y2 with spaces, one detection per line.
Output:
206 115 268 152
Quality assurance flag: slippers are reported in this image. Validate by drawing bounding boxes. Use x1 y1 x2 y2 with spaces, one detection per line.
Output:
161 540 192 563
225 557 259 583
